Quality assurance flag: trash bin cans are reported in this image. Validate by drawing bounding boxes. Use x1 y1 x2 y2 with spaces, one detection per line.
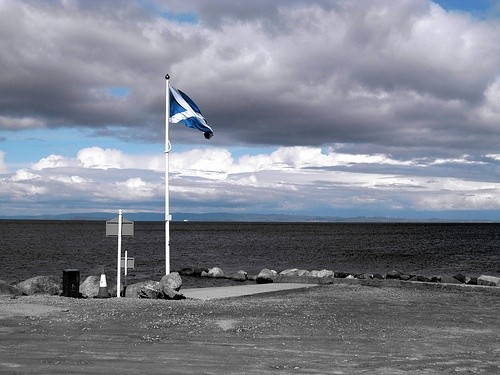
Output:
63 268 80 298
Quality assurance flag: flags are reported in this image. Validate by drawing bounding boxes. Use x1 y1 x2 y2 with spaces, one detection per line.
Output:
168 85 214 140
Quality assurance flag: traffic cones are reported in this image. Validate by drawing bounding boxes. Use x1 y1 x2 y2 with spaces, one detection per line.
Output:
93 265 111 299
